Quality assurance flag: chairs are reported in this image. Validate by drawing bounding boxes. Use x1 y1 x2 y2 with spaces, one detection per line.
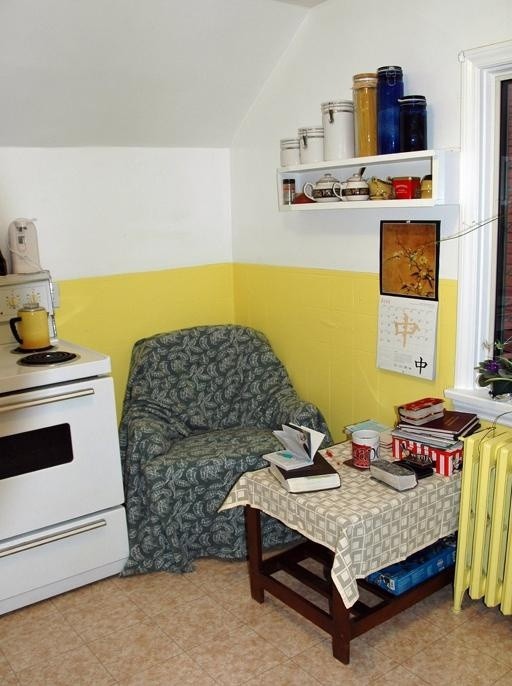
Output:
118 324 335 560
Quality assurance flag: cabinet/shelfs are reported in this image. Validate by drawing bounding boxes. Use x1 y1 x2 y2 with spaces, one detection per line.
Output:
277 148 445 210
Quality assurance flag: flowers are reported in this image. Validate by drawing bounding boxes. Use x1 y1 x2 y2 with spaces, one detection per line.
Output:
475 336 512 387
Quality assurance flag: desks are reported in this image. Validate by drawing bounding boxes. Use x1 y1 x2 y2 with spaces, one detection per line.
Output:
242 429 462 665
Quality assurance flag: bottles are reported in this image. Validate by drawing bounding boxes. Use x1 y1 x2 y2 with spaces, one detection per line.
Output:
282 179 296 204
375 65 405 155
297 126 323 162
398 95 427 152
351 72 379 157
420 175 432 199
321 98 354 161
280 138 298 166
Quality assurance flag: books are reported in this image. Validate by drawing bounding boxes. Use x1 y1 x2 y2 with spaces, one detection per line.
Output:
269 451 342 494
343 398 483 454
262 422 326 472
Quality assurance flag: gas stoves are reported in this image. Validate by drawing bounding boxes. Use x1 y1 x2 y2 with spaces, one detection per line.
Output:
0 340 108 393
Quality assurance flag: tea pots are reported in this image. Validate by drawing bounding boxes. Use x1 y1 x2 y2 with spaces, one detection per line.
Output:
302 173 341 202
10 303 52 350
333 173 370 201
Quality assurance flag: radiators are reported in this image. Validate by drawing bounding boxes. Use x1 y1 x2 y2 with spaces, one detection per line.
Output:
451 427 511 616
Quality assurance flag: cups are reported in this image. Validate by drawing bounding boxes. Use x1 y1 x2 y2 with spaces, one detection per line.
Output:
350 430 381 469
390 174 421 199
366 176 393 199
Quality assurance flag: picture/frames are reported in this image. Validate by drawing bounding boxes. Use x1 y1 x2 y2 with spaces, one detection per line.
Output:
378 218 442 300
